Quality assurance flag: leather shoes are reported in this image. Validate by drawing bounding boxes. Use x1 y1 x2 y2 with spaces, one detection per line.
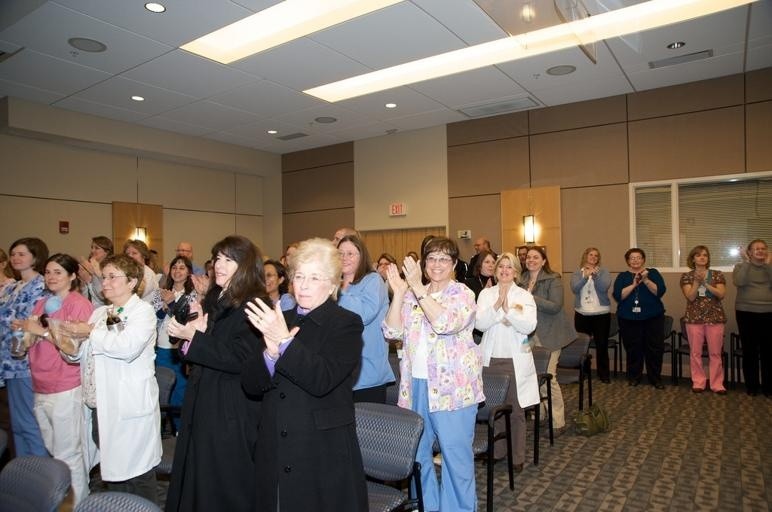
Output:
601 379 772 398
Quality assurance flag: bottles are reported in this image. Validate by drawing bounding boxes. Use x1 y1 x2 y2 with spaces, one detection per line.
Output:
105 308 124 332
8 324 25 357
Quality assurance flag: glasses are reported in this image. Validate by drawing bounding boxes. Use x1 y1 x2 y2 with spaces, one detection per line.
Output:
289 272 331 283
339 252 360 257
426 256 453 264
100 274 128 279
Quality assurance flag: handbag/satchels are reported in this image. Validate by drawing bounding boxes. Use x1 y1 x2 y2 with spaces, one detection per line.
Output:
572 403 611 435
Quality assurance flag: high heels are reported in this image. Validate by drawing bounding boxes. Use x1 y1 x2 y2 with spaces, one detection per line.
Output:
540 420 566 437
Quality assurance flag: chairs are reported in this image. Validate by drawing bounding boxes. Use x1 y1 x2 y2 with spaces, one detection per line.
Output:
588 313 742 388
156 365 427 511
525 332 593 466
1 455 161 511
384 366 516 511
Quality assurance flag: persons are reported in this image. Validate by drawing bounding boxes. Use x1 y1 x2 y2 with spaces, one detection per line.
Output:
0 228 579 512
731 238 772 397
612 248 668 389
681 244 730 395
569 246 612 384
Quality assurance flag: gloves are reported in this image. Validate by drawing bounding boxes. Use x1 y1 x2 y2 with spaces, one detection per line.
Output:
169 295 198 345
